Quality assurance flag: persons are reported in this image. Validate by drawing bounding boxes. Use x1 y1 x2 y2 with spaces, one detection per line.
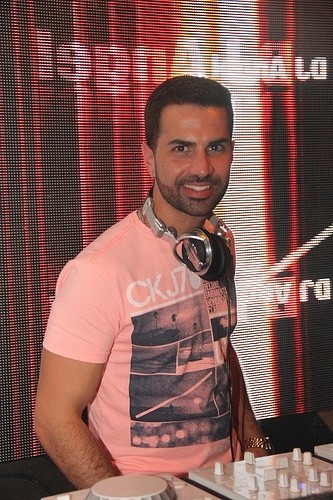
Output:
34 76 275 490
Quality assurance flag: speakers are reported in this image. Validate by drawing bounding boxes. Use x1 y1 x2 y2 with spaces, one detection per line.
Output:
0 454 77 500
257 411 333 455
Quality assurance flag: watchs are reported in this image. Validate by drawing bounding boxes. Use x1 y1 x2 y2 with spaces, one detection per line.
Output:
243 434 274 455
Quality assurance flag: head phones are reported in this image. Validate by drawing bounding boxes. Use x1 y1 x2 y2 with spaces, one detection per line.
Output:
141 187 232 282
